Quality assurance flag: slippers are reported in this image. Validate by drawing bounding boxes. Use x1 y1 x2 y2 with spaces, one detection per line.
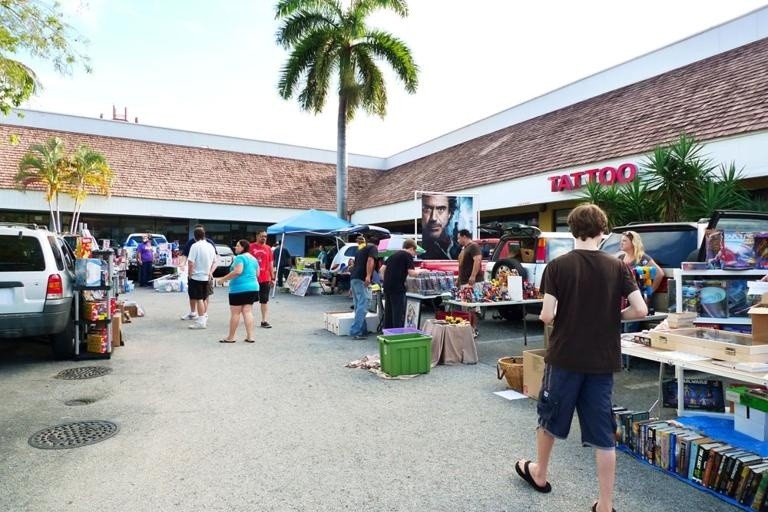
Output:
219 338 236 343
245 339 255 343
592 501 617 512
516 459 552 493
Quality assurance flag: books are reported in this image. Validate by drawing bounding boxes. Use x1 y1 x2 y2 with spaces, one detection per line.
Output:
611 406 768 512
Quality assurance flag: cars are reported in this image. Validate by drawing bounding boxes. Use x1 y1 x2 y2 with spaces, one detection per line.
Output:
597 221 709 309
209 243 235 277
325 232 433 286
697 209 768 263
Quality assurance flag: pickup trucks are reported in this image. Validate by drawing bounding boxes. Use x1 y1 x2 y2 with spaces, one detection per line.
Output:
422 233 532 326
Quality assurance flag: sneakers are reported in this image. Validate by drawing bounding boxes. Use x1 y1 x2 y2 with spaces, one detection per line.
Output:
261 321 272 328
182 313 208 329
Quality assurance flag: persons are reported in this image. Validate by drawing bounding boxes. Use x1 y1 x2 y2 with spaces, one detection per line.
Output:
349 236 380 339
187 226 217 329
423 195 462 259
136 236 154 287
218 239 260 342
381 240 428 328
271 240 328 287
615 231 665 365
454 230 482 338
248 230 275 328
515 204 649 512
181 224 218 320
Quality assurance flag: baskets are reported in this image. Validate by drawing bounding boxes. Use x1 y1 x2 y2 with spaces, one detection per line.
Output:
498 355 522 391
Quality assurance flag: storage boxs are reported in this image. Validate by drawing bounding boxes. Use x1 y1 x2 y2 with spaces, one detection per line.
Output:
323 311 378 336
747 292 768 346
522 348 545 400
733 403 768 441
383 327 421 335
125 305 137 317
376 332 433 377
112 312 121 347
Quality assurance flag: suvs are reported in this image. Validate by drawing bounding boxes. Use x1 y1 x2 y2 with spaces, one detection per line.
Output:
1 223 78 347
121 232 175 289
477 220 580 333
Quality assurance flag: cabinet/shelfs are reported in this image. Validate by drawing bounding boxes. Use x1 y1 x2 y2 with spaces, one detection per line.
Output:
73 250 114 358
673 267 768 416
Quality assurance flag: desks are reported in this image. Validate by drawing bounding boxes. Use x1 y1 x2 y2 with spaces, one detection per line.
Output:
284 267 325 295
621 332 768 419
442 298 543 346
405 291 452 313
